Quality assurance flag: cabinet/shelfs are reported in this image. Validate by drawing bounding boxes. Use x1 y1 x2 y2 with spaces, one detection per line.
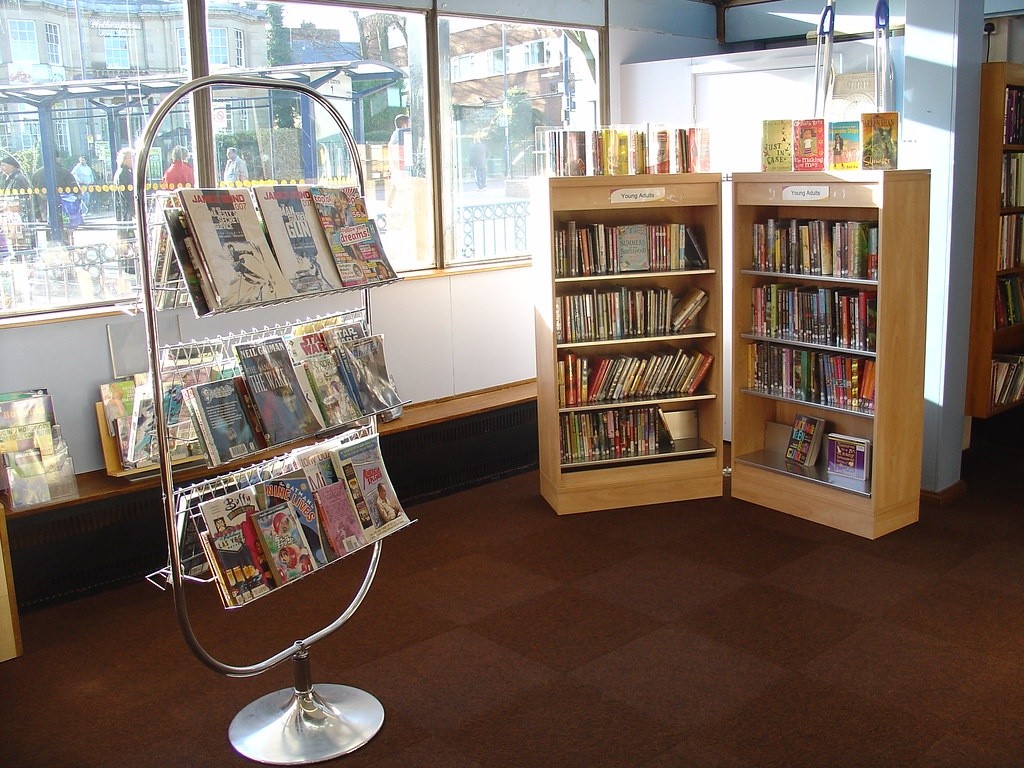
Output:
965 62 1024 419
730 170 934 542
528 175 724 515
128 75 418 768
0 423 78 512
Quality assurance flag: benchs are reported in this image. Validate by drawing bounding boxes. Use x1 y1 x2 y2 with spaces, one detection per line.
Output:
1 381 538 663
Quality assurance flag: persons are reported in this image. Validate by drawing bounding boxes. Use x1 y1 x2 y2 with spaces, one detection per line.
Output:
352 264 363 277
469 138 488 190
71 157 93 213
114 148 136 275
387 114 413 208
223 148 249 183
330 208 344 228
0 157 37 264
370 245 379 258
375 483 405 522
303 251 334 291
161 146 195 191
275 381 321 439
337 190 354 227
331 192 345 222
227 244 267 288
32 150 83 280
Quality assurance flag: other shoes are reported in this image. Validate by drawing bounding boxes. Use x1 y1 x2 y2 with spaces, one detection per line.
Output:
82 212 92 216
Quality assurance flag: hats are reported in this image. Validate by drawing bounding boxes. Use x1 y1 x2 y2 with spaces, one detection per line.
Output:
2 157 20 168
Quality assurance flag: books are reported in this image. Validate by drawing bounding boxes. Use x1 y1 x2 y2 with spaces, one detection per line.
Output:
0 388 71 506
749 218 878 483
101 186 410 607
554 221 715 464
760 111 898 171
547 123 709 180
990 87 1024 406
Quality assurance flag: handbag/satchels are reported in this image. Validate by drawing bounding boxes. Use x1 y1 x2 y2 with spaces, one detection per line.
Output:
59 193 84 228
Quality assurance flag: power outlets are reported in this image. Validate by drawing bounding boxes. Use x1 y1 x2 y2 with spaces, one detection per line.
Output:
984 18 996 35
